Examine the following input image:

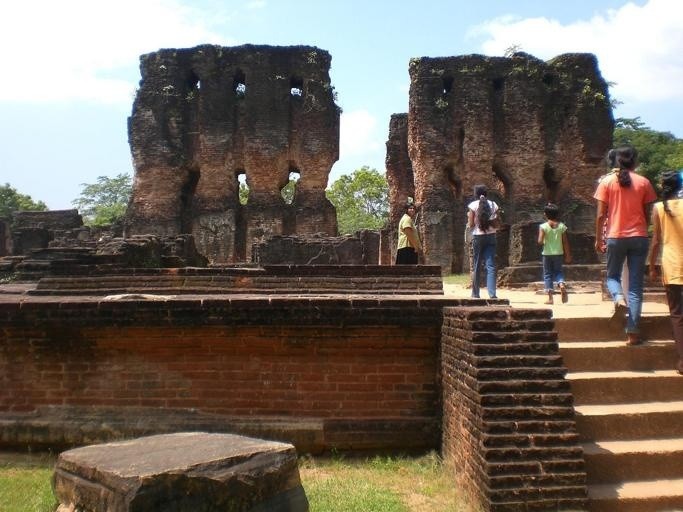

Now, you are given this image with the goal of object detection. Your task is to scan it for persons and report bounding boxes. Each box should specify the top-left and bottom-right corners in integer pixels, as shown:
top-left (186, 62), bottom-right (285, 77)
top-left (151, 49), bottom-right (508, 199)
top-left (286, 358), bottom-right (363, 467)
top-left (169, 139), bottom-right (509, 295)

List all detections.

top-left (592, 143), bottom-right (660, 344)
top-left (537, 202), bottom-right (571, 305)
top-left (396, 203), bottom-right (422, 264)
top-left (597, 149), bottom-right (629, 315)
top-left (467, 184), bottom-right (500, 298)
top-left (647, 171), bottom-right (682, 374)
